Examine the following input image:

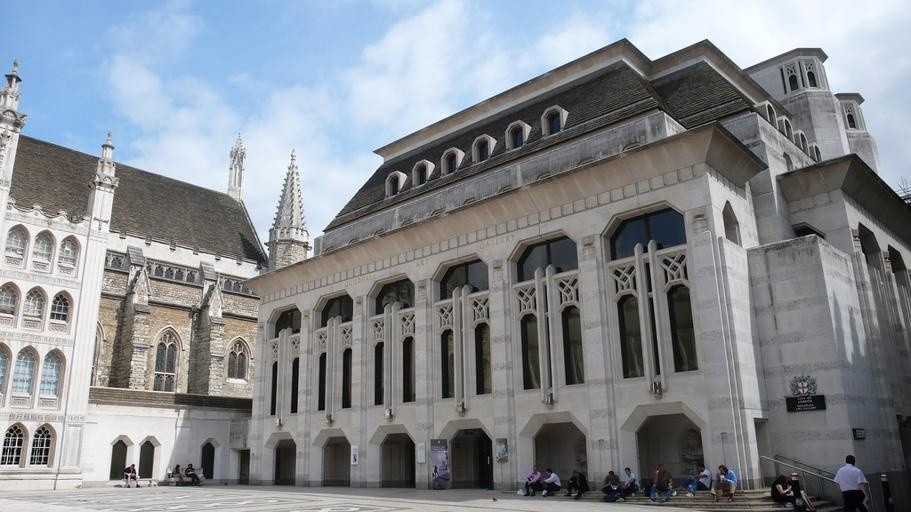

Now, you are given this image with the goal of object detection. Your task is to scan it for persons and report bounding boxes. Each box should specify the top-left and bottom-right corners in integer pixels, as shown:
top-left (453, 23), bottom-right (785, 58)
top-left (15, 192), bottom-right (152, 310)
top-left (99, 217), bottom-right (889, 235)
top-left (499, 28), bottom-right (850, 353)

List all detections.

top-left (833, 455), bottom-right (871, 511)
top-left (185, 463), bottom-right (204, 485)
top-left (172, 463), bottom-right (185, 485)
top-left (522, 461), bottom-right (813, 510)
top-left (124, 463), bottom-right (140, 487)
